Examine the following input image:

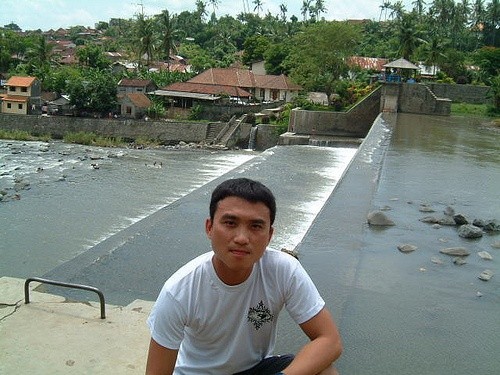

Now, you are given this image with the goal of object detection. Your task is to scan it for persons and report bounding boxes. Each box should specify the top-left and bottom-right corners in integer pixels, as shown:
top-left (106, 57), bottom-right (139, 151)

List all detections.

top-left (144, 178), bottom-right (342, 375)
